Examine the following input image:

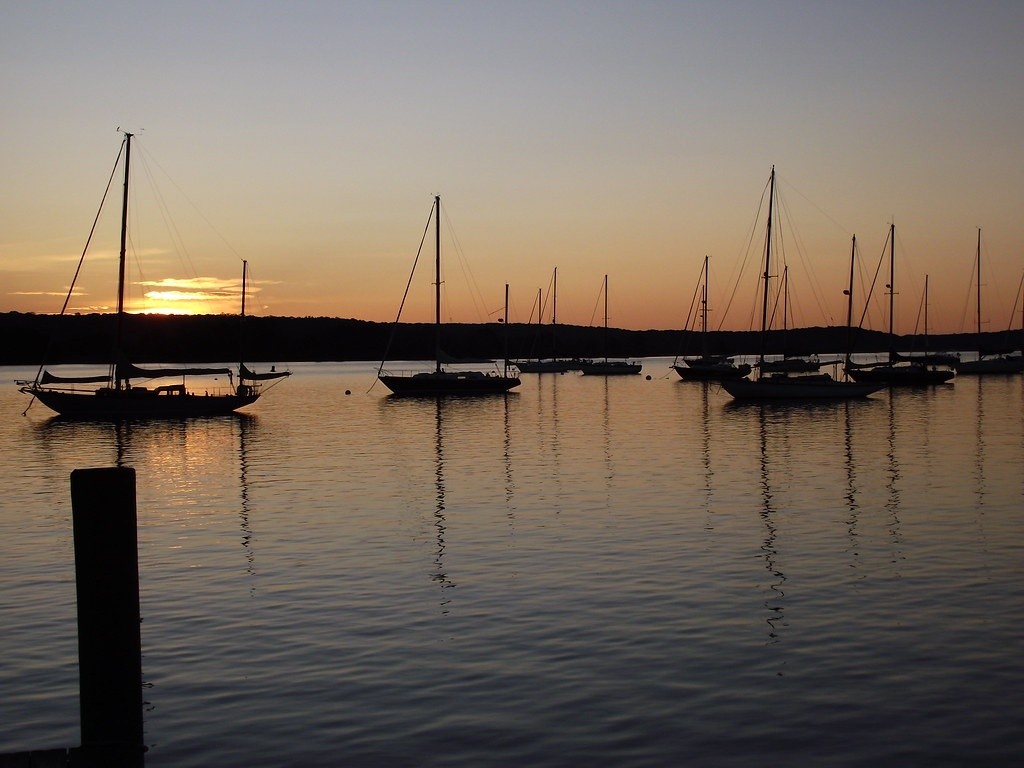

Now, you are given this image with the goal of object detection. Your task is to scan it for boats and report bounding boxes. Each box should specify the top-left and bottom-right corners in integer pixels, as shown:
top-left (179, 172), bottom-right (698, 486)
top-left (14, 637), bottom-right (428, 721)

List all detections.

top-left (513, 266), bottom-right (594, 373)
top-left (841, 224), bottom-right (957, 387)
top-left (38, 361), bottom-right (230, 385)
top-left (18, 128), bottom-right (263, 420)
top-left (952, 227), bottom-right (1024, 374)
top-left (668, 256), bottom-right (753, 381)
top-left (683, 286), bottom-right (734, 367)
top-left (889, 274), bottom-right (960, 365)
top-left (752, 264), bottom-right (822, 374)
top-left (846, 360), bottom-right (890, 371)
top-left (576, 274), bottom-right (643, 375)
top-left (373, 192), bottom-right (522, 398)
top-left (237, 360), bottom-right (293, 380)
top-left (714, 165), bottom-right (892, 401)
